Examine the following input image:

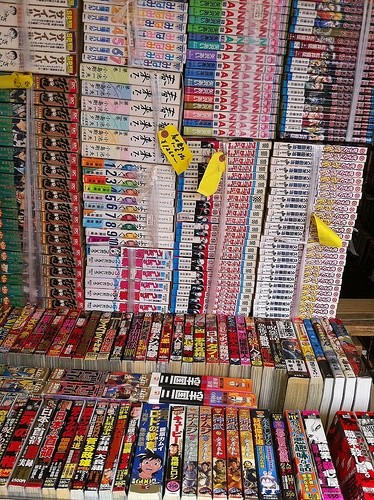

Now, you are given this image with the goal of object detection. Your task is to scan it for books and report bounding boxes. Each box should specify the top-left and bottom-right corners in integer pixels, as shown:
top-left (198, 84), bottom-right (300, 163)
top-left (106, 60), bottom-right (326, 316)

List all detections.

top-left (1, 1), bottom-right (374, 499)
